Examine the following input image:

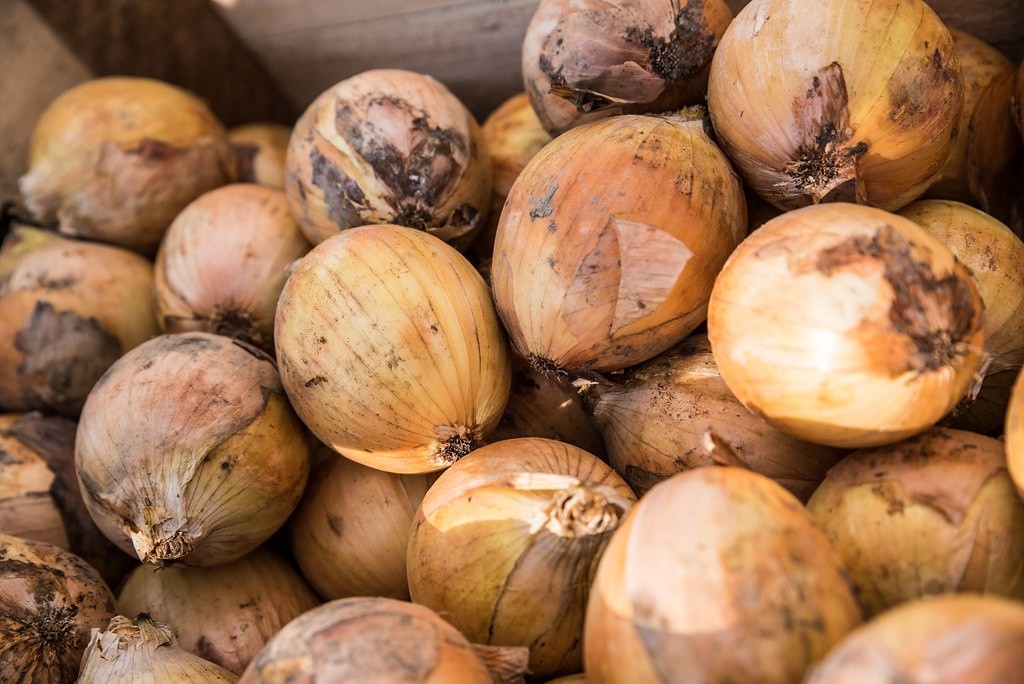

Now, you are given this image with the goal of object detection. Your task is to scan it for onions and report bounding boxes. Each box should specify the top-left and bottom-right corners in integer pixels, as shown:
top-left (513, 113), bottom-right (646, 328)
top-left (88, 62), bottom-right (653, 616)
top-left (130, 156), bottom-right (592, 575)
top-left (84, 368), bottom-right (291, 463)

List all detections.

top-left (0, 0), bottom-right (1024, 684)
